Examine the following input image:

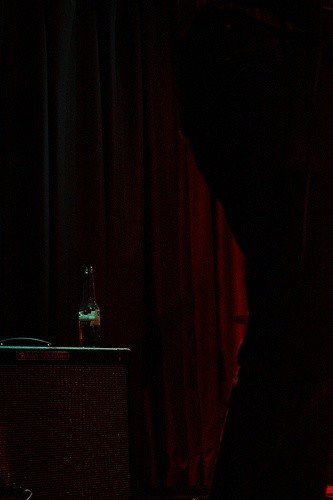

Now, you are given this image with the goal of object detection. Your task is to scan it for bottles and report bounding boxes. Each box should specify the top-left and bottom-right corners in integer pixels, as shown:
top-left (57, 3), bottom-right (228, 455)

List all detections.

top-left (78, 264), bottom-right (101, 348)
